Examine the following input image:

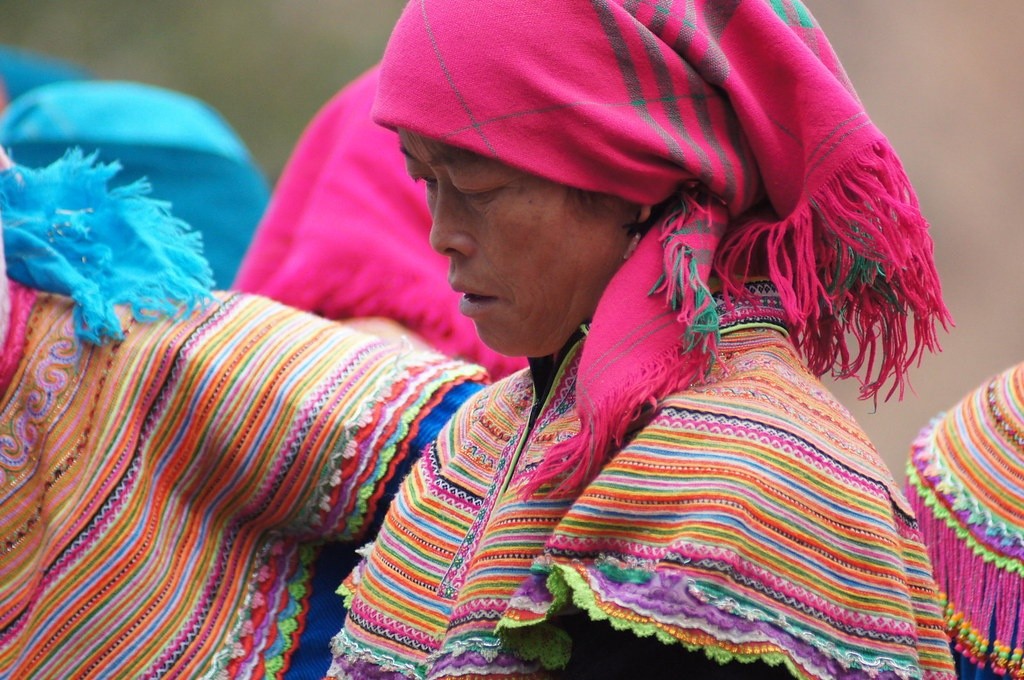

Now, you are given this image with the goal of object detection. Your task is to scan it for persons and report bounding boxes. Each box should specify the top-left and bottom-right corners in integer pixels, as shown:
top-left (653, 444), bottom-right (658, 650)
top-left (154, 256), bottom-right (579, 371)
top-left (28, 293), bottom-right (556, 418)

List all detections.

top-left (1, 1), bottom-right (1024, 678)
